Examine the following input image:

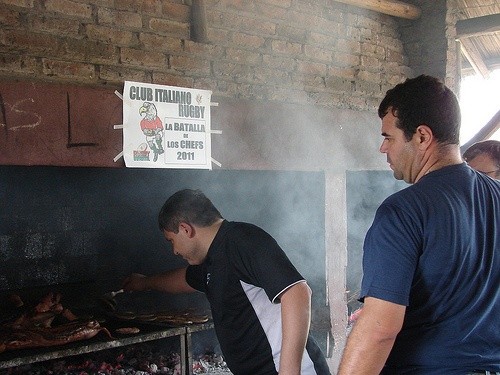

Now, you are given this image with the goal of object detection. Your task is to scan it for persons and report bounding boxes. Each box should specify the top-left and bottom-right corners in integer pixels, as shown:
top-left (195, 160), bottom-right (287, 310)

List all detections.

top-left (120, 188), bottom-right (331, 375)
top-left (337, 74), bottom-right (500, 375)
top-left (462, 140), bottom-right (500, 184)
